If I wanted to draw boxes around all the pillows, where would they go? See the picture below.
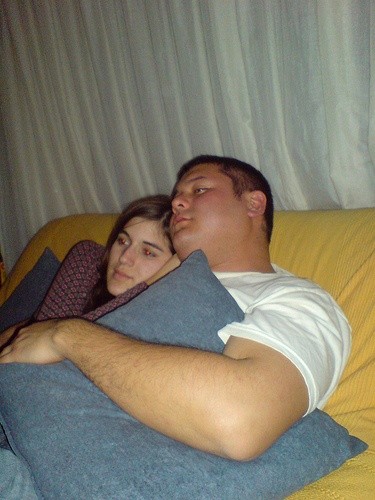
[0,249,369,500]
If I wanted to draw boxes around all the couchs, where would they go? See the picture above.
[1,206,374,498]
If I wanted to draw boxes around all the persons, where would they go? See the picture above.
[0,193,182,357]
[0,152,351,500]
